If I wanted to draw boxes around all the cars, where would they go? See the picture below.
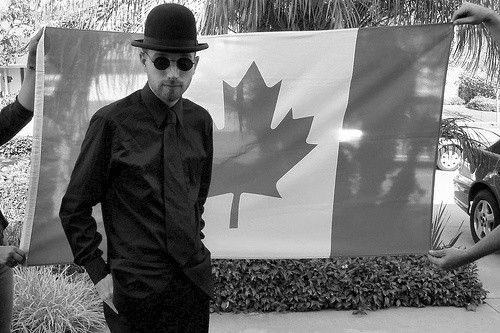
[433,111,500,172]
[452,136,499,244]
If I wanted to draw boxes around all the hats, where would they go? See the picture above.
[131,3,209,53]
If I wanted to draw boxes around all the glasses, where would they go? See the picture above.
[143,51,196,72]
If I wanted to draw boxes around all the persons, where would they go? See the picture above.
[429,2,500,270]
[0,27,45,333]
[60,3,214,333]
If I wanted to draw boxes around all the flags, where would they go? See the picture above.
[18,22,454,266]
